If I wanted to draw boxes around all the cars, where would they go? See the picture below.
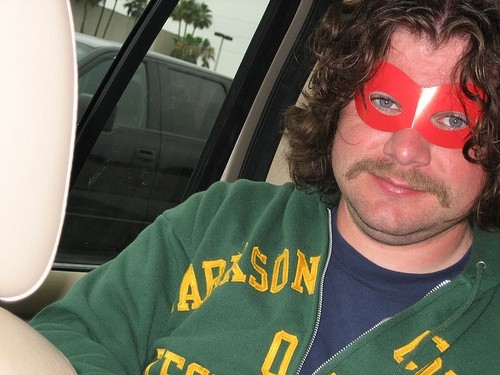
[54,29,236,269]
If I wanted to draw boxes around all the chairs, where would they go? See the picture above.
[0,0,80,374]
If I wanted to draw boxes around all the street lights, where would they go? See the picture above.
[213,32,233,71]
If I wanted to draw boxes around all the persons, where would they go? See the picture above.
[24,0,500,375]
